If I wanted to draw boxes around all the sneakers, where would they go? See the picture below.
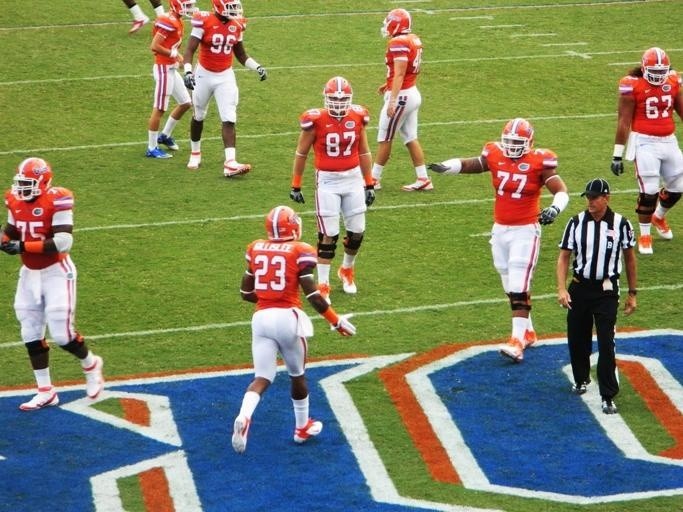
[289,417,326,447]
[570,379,590,395]
[127,16,150,35]
[315,281,333,308]
[186,151,202,170]
[497,327,539,365]
[16,384,62,411]
[649,212,677,241]
[221,158,252,178]
[228,412,252,456]
[600,396,619,415]
[360,175,383,191]
[399,176,435,193]
[635,233,654,256]
[79,354,108,401]
[144,146,174,161]
[156,131,180,151]
[336,264,359,296]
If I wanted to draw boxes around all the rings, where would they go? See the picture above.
[560,304,563,307]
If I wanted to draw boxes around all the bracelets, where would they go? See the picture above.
[365,175,374,186]
[245,56,261,70]
[2,235,10,245]
[170,49,177,59]
[551,191,570,213]
[292,175,302,188]
[439,158,462,176]
[23,241,43,254]
[628,290,637,295]
[321,307,339,326]
[184,63,193,76]
[612,144,626,158]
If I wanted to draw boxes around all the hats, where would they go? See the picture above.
[578,177,611,199]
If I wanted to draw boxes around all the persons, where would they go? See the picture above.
[123,0,164,34]
[183,1,268,178]
[555,179,638,414]
[232,206,356,454]
[0,157,104,412]
[611,47,683,256]
[144,1,195,159]
[289,75,375,306]
[364,9,435,192]
[428,118,570,361]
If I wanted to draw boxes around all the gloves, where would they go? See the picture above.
[255,64,268,82]
[608,155,628,179]
[535,204,561,229]
[286,186,307,205]
[329,315,357,337]
[426,159,452,178]
[175,51,183,67]
[0,235,24,256]
[364,184,376,207]
[183,71,196,91]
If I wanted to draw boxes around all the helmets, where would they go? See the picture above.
[17,156,54,197]
[640,46,672,79]
[263,204,302,243]
[381,7,414,37]
[500,116,537,154]
[320,75,355,100]
[210,0,241,17]
[168,0,196,17]
